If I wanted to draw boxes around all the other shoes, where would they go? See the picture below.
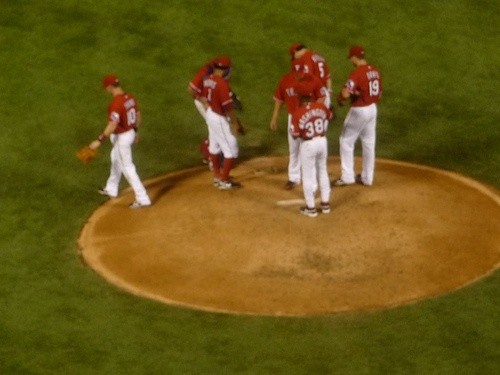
[214,178,241,189]
[334,178,347,185]
[301,205,319,217]
[359,174,362,180]
[130,203,148,208]
[286,181,293,190]
[321,204,330,214]
[99,189,118,198]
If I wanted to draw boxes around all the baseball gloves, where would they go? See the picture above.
[75,145,97,163]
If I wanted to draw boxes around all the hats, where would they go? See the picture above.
[292,59,311,77]
[103,76,118,89]
[216,55,231,68]
[289,43,303,62]
[348,48,365,59]
[298,82,314,96]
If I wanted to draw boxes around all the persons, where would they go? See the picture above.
[77,76,151,210]
[337,46,382,186]
[188,55,248,190]
[271,43,333,217]
